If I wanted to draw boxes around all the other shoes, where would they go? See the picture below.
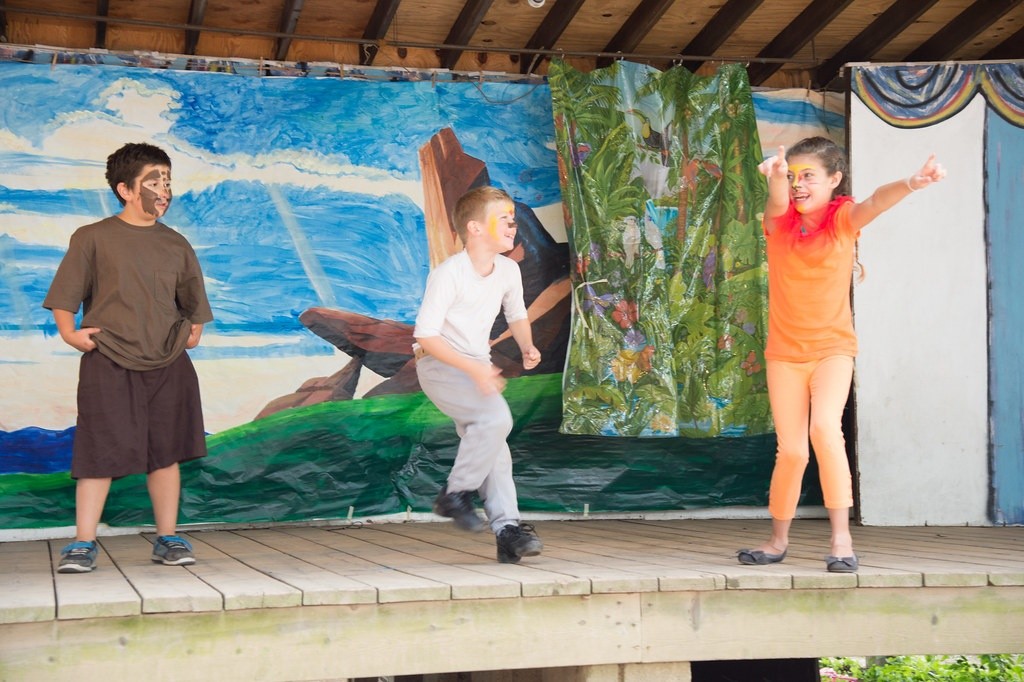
[736,546,787,565]
[824,552,859,573]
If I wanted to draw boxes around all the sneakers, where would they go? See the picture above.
[435,485,486,534]
[57,541,97,573]
[496,523,544,563]
[151,535,196,565]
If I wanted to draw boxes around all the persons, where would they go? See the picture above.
[737,135,946,572]
[412,186,541,564]
[42,143,215,573]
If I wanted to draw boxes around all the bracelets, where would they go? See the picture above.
[907,174,927,195]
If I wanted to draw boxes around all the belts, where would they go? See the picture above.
[414,347,430,360]
